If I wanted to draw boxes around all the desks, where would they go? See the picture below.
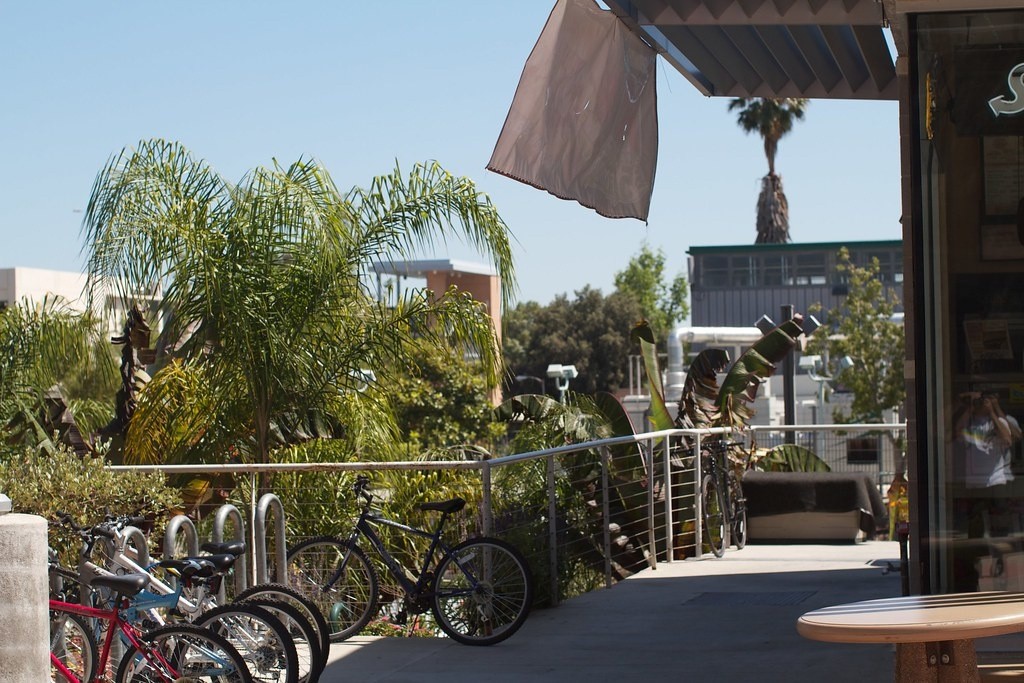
[797,591,1024,683]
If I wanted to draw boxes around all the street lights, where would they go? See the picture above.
[754,303,822,444]
[546,364,579,406]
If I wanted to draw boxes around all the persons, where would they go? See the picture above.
[955,390,1023,564]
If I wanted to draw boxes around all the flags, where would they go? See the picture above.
[485,0,658,227]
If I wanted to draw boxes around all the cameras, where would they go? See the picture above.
[973,397,983,406]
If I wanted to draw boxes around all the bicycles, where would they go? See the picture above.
[47,509,331,683]
[670,439,747,558]
[269,473,534,647]
